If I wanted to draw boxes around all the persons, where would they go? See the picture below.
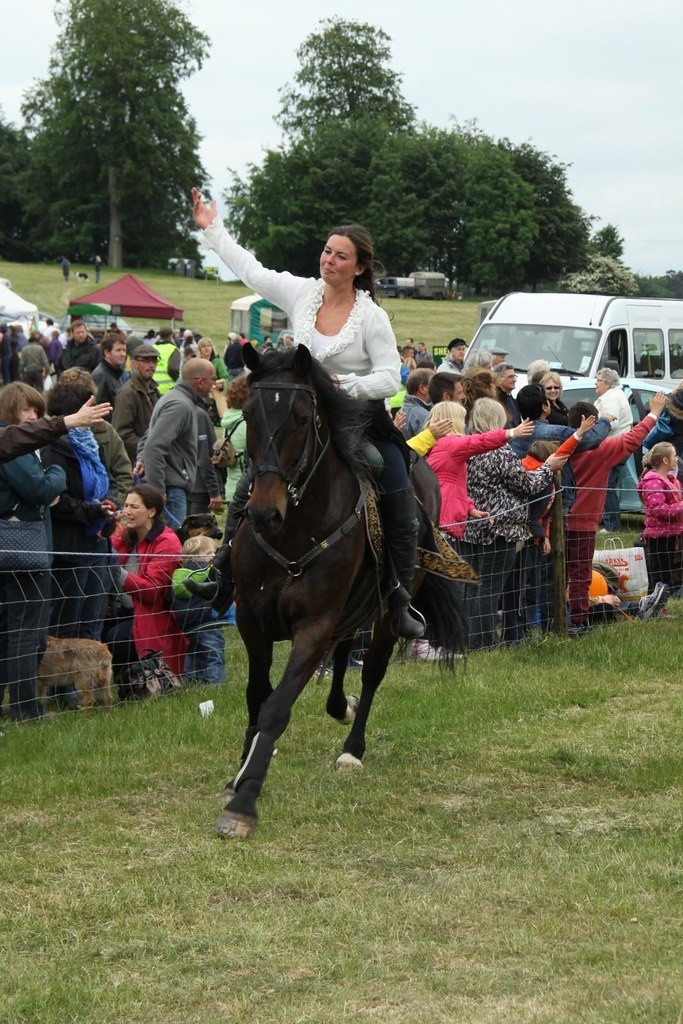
[95,254,101,283]
[0,316,683,722]
[182,184,426,639]
[60,258,70,280]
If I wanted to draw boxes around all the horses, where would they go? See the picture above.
[214,341,443,841]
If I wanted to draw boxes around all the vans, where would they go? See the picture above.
[408,272,446,299]
[460,292,683,387]
[374,278,415,300]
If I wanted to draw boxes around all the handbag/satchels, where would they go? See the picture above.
[592,537,649,602]
[641,404,672,450]
[0,519,49,569]
[119,648,181,700]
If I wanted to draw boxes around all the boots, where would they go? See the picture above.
[379,485,425,638]
[183,493,249,618]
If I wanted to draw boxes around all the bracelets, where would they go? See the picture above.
[510,427,515,440]
[573,432,582,441]
[648,412,658,421]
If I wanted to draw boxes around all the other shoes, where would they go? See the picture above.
[1,707,10,715]
[598,527,619,534]
[42,709,56,722]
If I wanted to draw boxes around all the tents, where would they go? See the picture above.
[66,274,184,329]
[230,292,294,350]
[0,277,38,316]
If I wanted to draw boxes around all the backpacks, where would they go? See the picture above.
[211,419,248,470]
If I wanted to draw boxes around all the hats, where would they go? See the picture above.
[447,339,465,351]
[488,347,509,355]
[132,344,160,358]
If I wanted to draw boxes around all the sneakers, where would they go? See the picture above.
[431,643,466,659]
[332,655,364,669]
[316,652,331,676]
[638,581,670,622]
[406,637,443,661]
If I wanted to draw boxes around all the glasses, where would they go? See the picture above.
[134,357,157,363]
[194,376,216,382]
[545,386,560,390]
[506,374,517,379]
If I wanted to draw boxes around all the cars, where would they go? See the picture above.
[0,312,134,344]
[556,378,672,514]
